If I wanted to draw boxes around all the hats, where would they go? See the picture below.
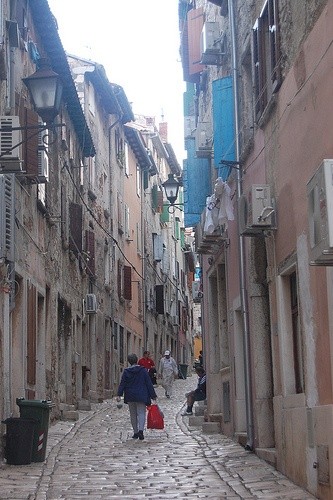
[164,350,170,355]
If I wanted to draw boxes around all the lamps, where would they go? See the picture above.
[163,173,183,213]
[0,55,65,156]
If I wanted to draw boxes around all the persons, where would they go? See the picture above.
[198,350,203,364]
[117,354,158,440]
[158,350,178,398]
[138,350,155,371]
[180,367,206,416]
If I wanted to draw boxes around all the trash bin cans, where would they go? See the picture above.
[1,418,40,464]
[195,363,200,368]
[16,396,50,462]
[178,364,188,379]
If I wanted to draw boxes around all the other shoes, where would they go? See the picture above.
[181,411,193,416]
[165,391,170,398]
[132,433,139,439]
[138,430,144,440]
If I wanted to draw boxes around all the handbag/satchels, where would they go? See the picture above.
[147,404,164,429]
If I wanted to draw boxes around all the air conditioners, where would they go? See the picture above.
[38,120,49,153]
[194,121,212,158]
[86,293,97,313]
[171,315,179,325]
[195,214,223,255]
[193,281,199,303]
[0,115,23,171]
[239,184,274,235]
[38,150,49,182]
[200,21,220,64]
[305,159,333,267]
